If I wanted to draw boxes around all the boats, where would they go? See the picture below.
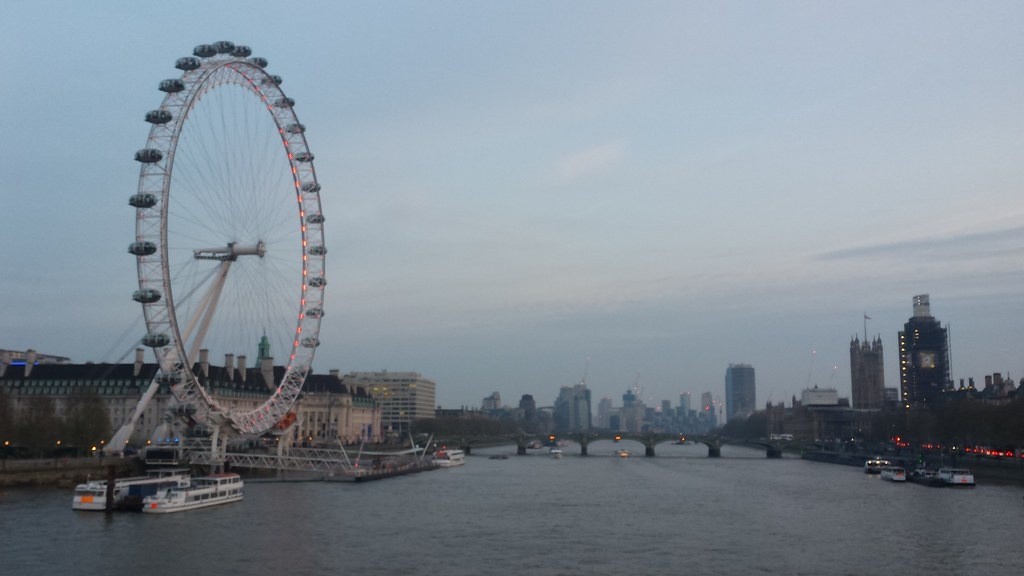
[617,449,631,458]
[142,474,245,513]
[549,447,562,458]
[908,467,977,487]
[864,457,891,473]
[430,449,464,467]
[71,468,191,510]
[880,463,906,482]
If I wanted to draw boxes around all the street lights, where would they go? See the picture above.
[385,392,396,432]
